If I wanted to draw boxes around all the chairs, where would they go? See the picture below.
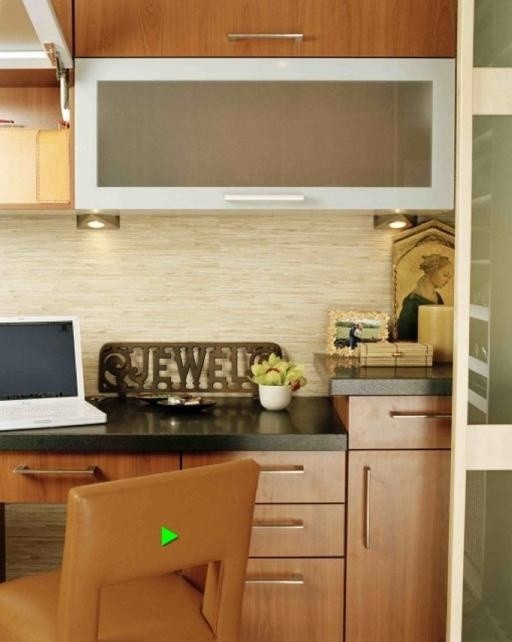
[0,459,261,641]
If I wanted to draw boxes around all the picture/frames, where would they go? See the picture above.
[327,309,389,357]
[392,218,455,341]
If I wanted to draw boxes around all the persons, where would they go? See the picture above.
[349,324,363,348]
[398,253,453,340]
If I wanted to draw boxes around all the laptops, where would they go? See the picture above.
[0,316,107,431]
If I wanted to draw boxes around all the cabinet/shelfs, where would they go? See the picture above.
[0,452,182,504]
[1,2,457,214]
[333,394,452,641]
[181,451,346,641]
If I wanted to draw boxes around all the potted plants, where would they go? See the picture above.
[248,352,308,411]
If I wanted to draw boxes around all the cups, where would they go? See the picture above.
[259,413,289,432]
[148,394,212,410]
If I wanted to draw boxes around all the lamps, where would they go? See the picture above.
[373,214,417,231]
[77,214,121,230]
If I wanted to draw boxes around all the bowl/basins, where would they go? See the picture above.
[259,385,293,410]
